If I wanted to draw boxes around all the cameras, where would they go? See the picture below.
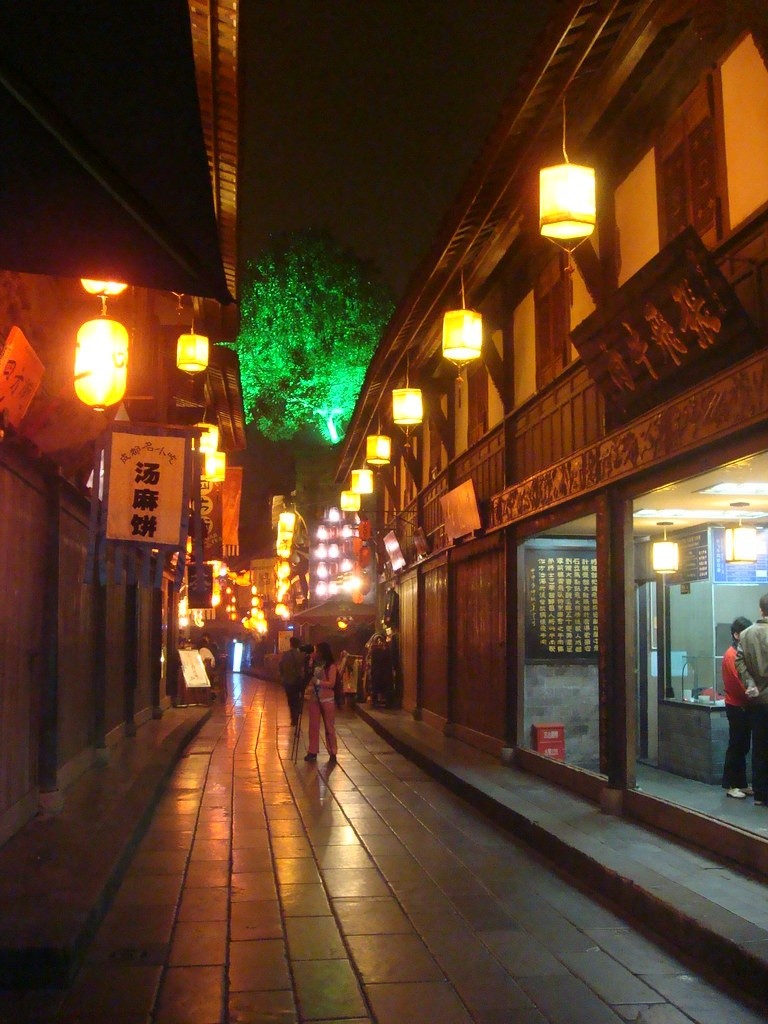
[300,644,314,654]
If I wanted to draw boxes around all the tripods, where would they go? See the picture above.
[291,654,336,765]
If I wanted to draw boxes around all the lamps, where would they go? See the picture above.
[441,270,483,406]
[350,470,373,506]
[366,411,391,485]
[652,522,680,575]
[393,354,423,474]
[725,504,759,565]
[340,490,361,523]
[176,318,208,377]
[191,407,220,454]
[539,94,595,306]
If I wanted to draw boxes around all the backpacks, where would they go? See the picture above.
[326,664,346,706]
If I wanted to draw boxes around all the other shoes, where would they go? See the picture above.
[754,801,764,805]
[330,754,337,762]
[726,786,753,799]
[303,752,318,762]
[290,720,298,726]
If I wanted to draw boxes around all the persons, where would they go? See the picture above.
[721,592,768,806]
[279,637,305,726]
[304,642,337,764]
[199,632,219,668]
